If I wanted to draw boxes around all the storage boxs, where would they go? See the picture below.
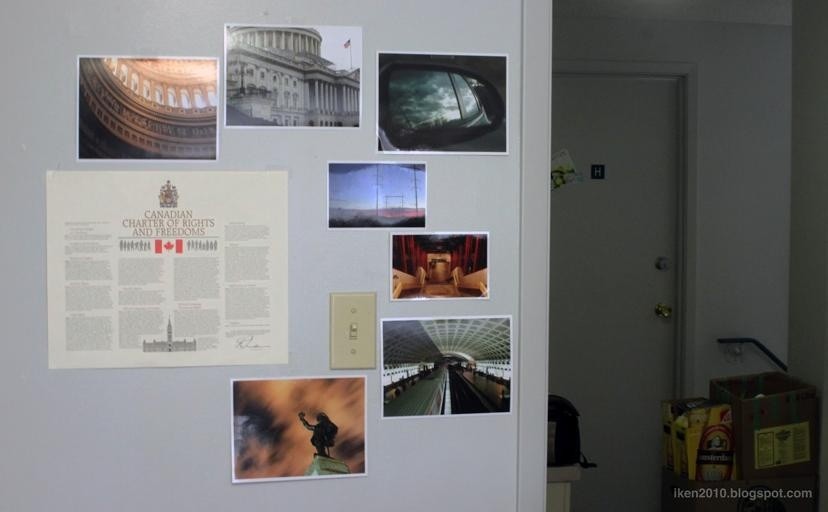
[707,371,821,481]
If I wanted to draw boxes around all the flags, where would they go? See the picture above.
[344,40,351,50]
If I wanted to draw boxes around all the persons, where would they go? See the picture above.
[299,411,338,457]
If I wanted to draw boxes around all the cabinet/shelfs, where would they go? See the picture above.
[546,464,580,512]
